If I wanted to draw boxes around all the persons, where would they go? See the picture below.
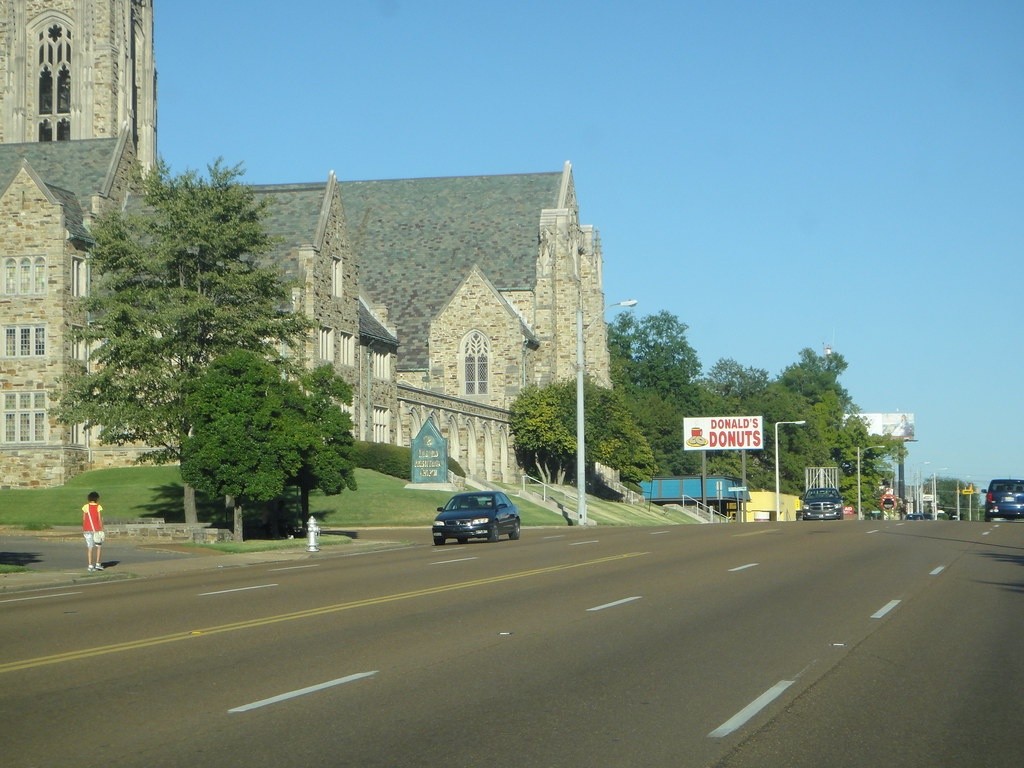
[79,492,106,573]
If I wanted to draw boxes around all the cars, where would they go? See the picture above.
[923,513,934,520]
[799,488,845,520]
[981,479,1024,522]
[432,491,520,545]
[905,514,924,520]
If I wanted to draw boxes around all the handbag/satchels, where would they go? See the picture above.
[93,531,105,543]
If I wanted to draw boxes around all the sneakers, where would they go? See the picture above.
[95,563,104,571]
[87,564,98,573]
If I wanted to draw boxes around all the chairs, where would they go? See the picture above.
[460,498,478,509]
[997,486,1003,492]
[1016,487,1023,492]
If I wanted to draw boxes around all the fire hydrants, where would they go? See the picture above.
[307,516,321,552]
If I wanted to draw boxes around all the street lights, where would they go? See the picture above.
[577,300,638,526]
[775,420,806,522]
[857,446,885,520]
[909,462,930,513]
